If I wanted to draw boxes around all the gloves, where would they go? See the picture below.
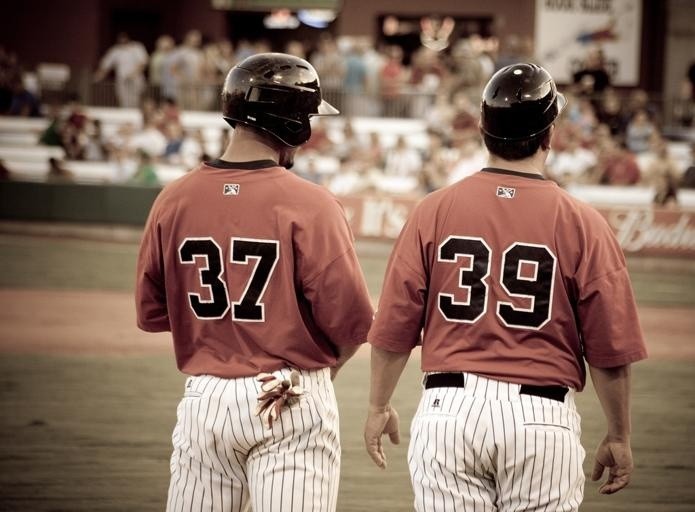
[254,371,302,430]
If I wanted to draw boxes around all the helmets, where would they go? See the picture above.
[480,64,566,139]
[222,53,340,147]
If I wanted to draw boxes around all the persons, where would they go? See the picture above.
[132,51,377,511]
[364,61,649,512]
